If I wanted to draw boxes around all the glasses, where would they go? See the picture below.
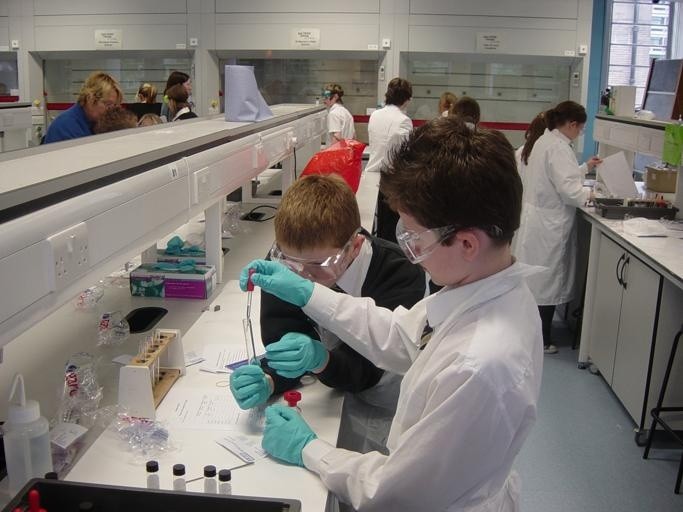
[270,227,367,285]
[395,217,459,265]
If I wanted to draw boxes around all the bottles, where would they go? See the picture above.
[143,460,232,497]
[2,370,52,499]
[244,317,256,369]
[135,329,160,391]
[315,98,319,105]
[599,89,610,114]
[621,193,673,210]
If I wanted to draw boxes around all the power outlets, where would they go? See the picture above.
[44,217,93,290]
[285,131,296,154]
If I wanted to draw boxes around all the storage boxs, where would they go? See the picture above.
[642,166,678,195]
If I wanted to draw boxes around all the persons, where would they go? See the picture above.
[90,70,197,132]
[447,96,480,127]
[320,81,356,147]
[239,114,545,512]
[511,99,602,355]
[41,70,122,143]
[438,92,456,116]
[228,172,426,412]
[363,77,414,173]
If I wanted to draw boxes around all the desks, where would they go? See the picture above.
[587,202,683,446]
[0,139,386,512]
[573,193,594,221]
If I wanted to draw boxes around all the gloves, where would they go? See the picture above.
[229,365,272,410]
[240,259,315,308]
[264,331,328,379]
[262,403,320,467]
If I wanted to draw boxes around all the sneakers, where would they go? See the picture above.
[544,344,557,354]
